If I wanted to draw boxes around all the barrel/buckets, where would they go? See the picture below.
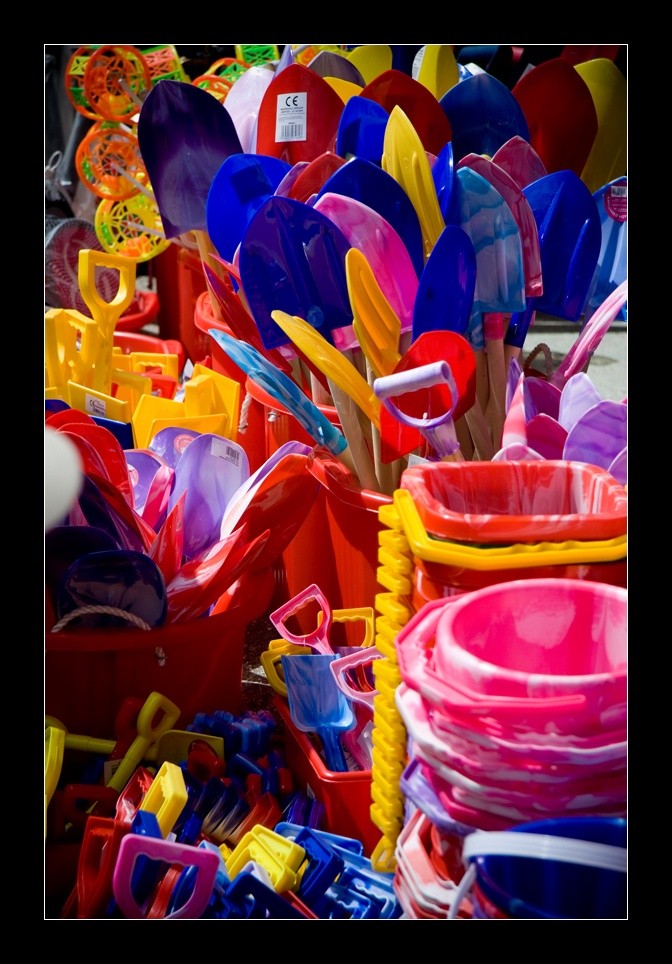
[45,291,627,921]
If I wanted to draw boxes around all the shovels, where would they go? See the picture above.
[134,43,630,501]
[44,402,323,635]
[280,652,357,774]
